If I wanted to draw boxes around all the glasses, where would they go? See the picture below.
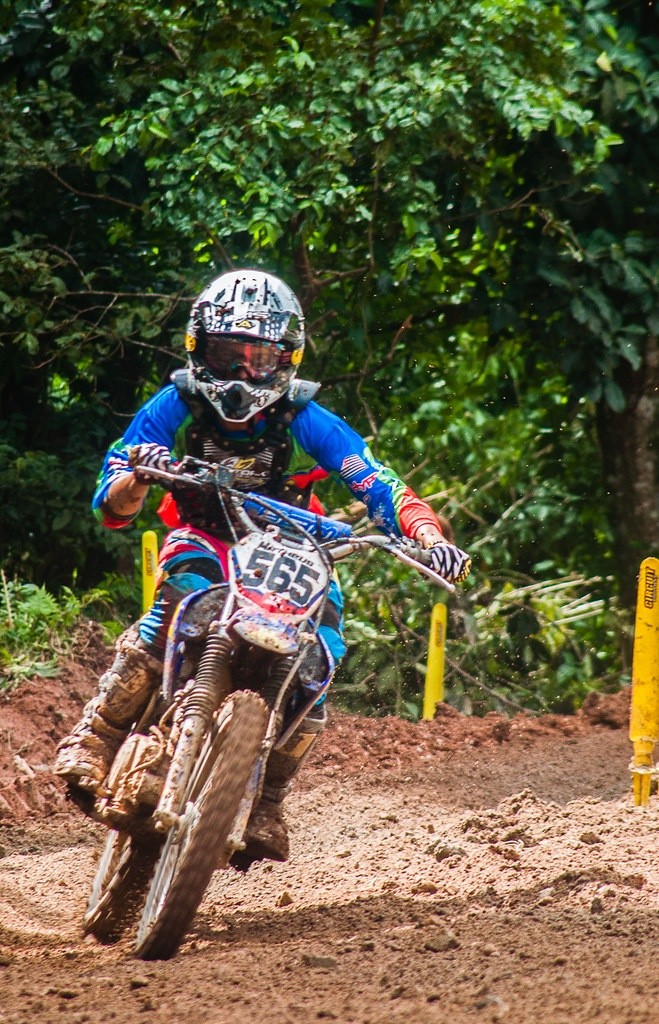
[184,334,303,383]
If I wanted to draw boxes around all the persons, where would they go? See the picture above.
[55,268,476,861]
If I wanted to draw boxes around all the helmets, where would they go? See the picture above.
[183,270,306,422]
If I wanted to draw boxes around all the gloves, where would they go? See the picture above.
[127,443,172,484]
[420,542,472,584]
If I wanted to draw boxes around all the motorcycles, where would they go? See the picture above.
[62,447,455,959]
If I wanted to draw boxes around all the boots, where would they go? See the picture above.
[53,620,164,787]
[241,705,327,862]
